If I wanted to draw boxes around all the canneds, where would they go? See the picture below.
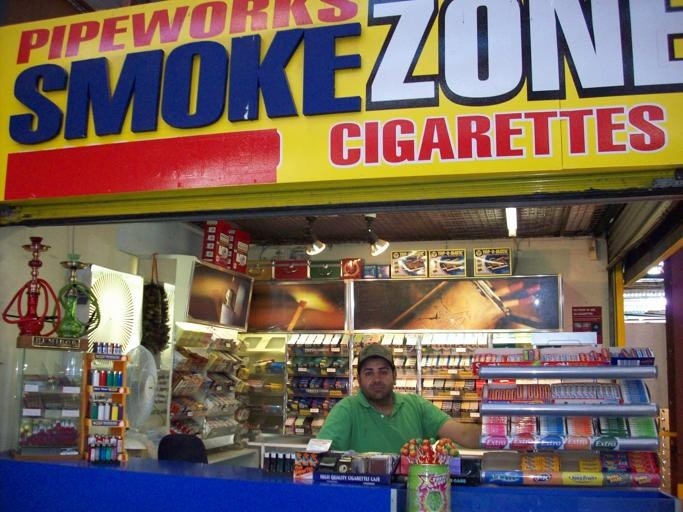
[94,342,121,355]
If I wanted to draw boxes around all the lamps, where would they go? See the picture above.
[301,212,326,255]
[363,213,390,257]
[504,205,518,238]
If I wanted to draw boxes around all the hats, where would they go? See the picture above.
[357,342,395,368]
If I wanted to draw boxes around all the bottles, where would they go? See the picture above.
[218,287,235,323]
[87,368,123,463]
[90,340,121,355]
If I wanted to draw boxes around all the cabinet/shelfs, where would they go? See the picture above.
[13,348,130,471]
[172,323,244,450]
[474,360,663,485]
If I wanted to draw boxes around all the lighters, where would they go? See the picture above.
[86,341,127,464]
[264,454,296,474]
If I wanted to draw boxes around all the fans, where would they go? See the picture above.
[122,345,158,449]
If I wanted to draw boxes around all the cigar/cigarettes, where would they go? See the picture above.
[498,281,544,308]
[286,300,309,333]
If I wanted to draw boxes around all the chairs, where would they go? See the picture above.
[157,431,211,464]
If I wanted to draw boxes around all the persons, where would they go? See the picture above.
[315,343,482,456]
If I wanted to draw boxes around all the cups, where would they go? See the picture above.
[386,277,508,331]
